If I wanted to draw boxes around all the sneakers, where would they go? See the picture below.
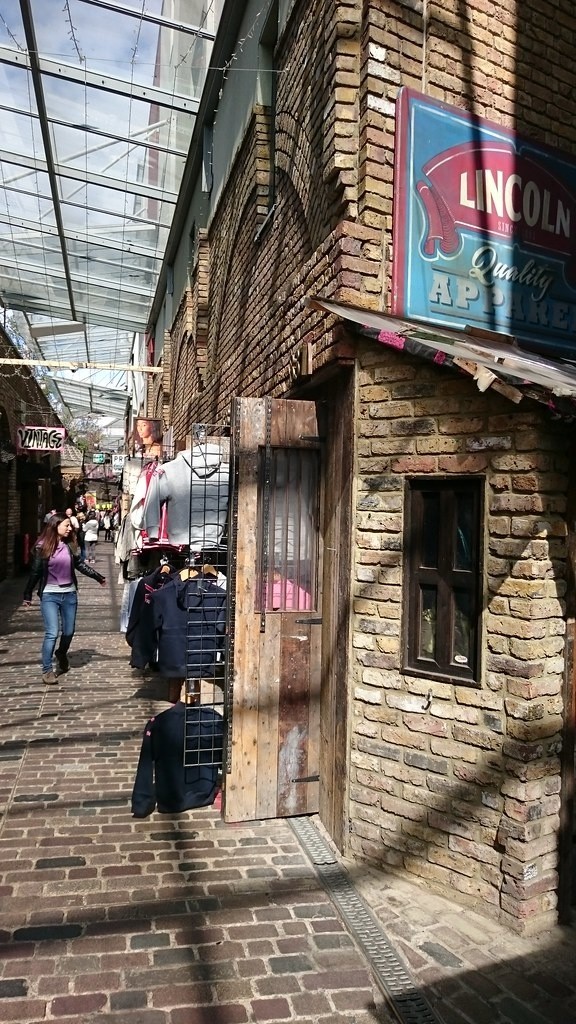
[56,650,70,670]
[43,671,59,684]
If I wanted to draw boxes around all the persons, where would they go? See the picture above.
[22,510,107,683]
[64,503,122,563]
[135,419,161,458]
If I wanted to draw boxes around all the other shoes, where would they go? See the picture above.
[84,559,89,563]
[90,560,95,564]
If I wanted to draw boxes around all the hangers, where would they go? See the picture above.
[180,694,196,704]
[198,554,220,580]
[179,554,199,583]
[159,555,170,575]
